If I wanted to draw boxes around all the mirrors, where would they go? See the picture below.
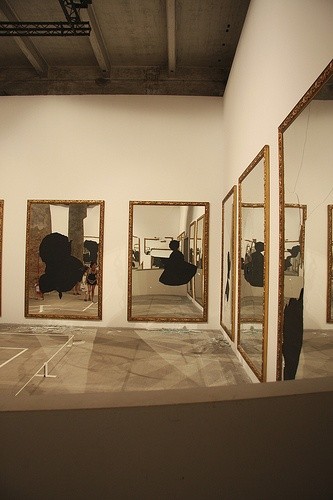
[219,184,235,343]
[275,56,332,382]
[236,144,268,384]
[240,202,264,324]
[283,203,307,301]
[132,213,204,310]
[23,199,104,321]
[127,200,210,323]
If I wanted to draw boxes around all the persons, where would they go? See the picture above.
[71,279,81,296]
[85,261,99,301]
